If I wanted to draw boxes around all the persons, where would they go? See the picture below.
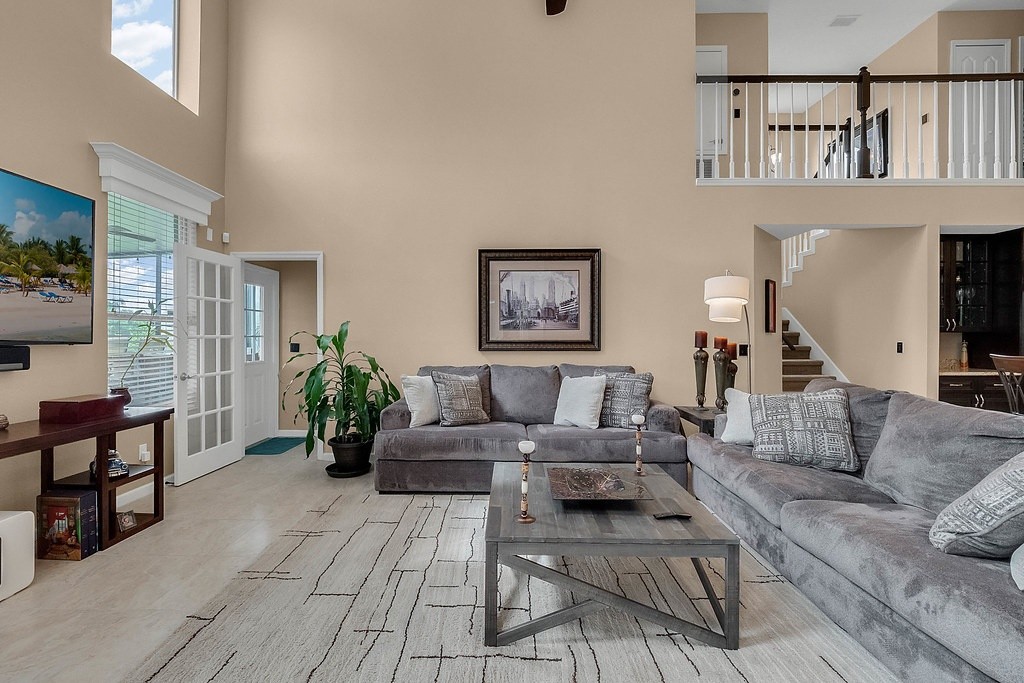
[62,276,67,283]
[50,277,54,283]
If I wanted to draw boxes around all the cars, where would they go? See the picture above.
[43,279,54,284]
[0,278,11,284]
[89,452,129,478]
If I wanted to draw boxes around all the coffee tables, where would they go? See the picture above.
[484,460,745,651]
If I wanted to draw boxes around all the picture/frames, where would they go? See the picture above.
[477,248,602,351]
[765,278,777,333]
[115,510,138,533]
[826,109,890,178]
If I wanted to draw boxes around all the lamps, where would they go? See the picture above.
[704,270,752,394]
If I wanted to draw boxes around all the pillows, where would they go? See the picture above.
[594,369,655,430]
[401,373,440,428]
[721,387,756,445]
[430,368,491,426]
[552,375,607,428]
[930,450,1024,559]
[748,387,861,471]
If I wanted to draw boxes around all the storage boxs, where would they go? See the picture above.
[0,508,36,603]
[34,493,97,561]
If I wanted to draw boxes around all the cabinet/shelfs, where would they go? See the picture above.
[0,404,177,548]
[939,374,1024,412]
[940,236,994,334]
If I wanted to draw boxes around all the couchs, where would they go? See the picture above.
[372,363,692,494]
[685,379,1024,683]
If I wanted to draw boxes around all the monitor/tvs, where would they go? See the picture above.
[0,169,96,344]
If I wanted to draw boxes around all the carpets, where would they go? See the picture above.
[245,436,307,456]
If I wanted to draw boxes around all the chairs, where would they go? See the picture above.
[990,352,1024,417]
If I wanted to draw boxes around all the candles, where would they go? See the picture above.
[694,329,708,347]
[725,341,737,358]
[713,335,727,347]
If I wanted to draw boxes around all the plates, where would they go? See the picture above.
[548,467,654,500]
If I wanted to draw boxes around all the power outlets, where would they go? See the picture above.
[138,443,148,460]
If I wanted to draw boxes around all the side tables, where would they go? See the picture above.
[674,404,727,496]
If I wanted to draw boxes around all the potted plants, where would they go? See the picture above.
[111,292,187,406]
[276,319,400,467]
